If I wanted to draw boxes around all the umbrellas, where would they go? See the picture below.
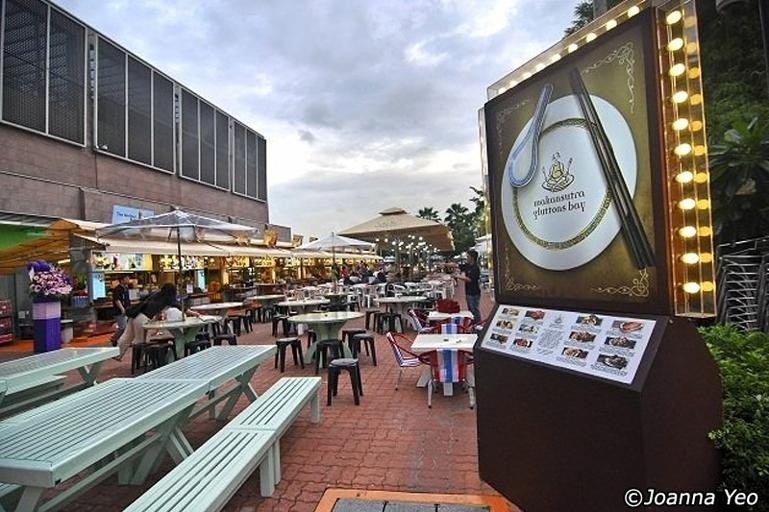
[291,234,372,283]
[336,207,449,280]
[426,231,454,244]
[438,241,455,251]
[454,253,468,260]
[95,209,260,320]
[475,234,491,242]
[470,243,485,254]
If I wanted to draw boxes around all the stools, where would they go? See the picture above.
[150,337,175,344]
[312,339,345,376]
[219,376,324,485]
[308,329,319,348]
[272,314,290,337]
[352,333,378,366]
[224,317,242,335]
[186,341,211,358]
[274,338,303,373]
[252,304,263,323]
[214,334,238,345]
[327,358,363,407]
[382,313,404,334]
[195,332,209,339]
[365,310,380,330]
[130,342,148,374]
[237,314,253,333]
[374,312,385,332]
[263,305,276,324]
[343,328,366,351]
[150,344,177,369]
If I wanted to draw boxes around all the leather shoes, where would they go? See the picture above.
[111,339,116,346]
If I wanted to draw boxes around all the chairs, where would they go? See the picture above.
[318,293,349,309]
[427,348,476,411]
[387,332,430,391]
[430,324,470,334]
[409,309,436,334]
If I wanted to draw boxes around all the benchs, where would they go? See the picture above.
[120,429,274,512]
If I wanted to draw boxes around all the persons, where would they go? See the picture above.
[450,249,482,325]
[359,264,369,274]
[341,266,350,278]
[361,269,374,283]
[331,263,340,283]
[342,258,348,266]
[377,268,386,283]
[343,276,355,291]
[111,282,200,362]
[109,275,131,347]
[357,260,365,271]
